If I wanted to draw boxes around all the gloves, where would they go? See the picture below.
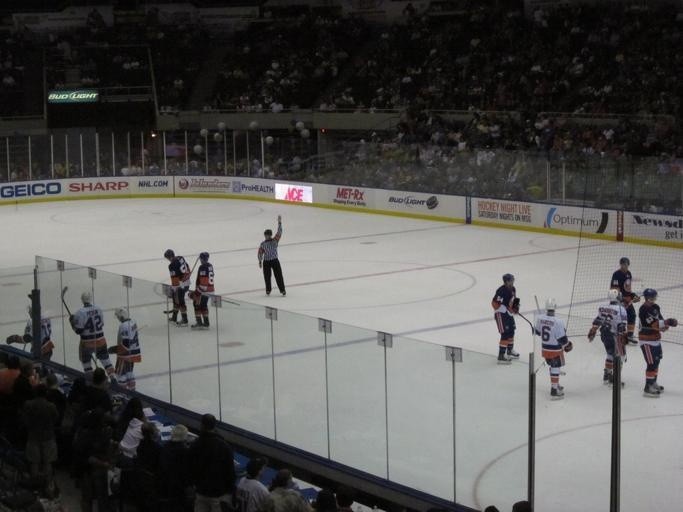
[588,327,596,342]
[658,317,678,331]
[564,341,572,352]
[6,334,20,344]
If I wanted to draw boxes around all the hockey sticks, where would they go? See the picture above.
[163,256,199,314]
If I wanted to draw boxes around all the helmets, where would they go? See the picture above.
[641,288,657,297]
[115,305,129,320]
[620,257,630,265]
[544,296,557,310]
[164,249,175,257]
[264,229,272,236]
[502,273,514,281]
[81,291,92,303]
[200,252,209,259]
[607,288,618,299]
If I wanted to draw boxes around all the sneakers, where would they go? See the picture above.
[608,368,624,387]
[110,374,118,383]
[557,384,564,391]
[176,314,188,324]
[191,320,202,328]
[654,378,664,393]
[496,346,512,361]
[550,387,565,396]
[507,344,520,356]
[644,379,660,394]
[603,370,613,382]
[266,288,271,293]
[281,289,286,295]
[169,311,177,322]
[202,317,210,328]
[628,332,637,344]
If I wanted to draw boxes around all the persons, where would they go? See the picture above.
[608,256,638,344]
[0,0,681,214]
[492,273,524,363]
[638,287,678,396]
[257,215,288,297]
[588,287,627,385]
[0,346,535,512]
[163,247,191,326]
[188,252,215,328]
[106,305,141,392]
[535,297,572,397]
[68,288,116,383]
[4,302,53,358]
